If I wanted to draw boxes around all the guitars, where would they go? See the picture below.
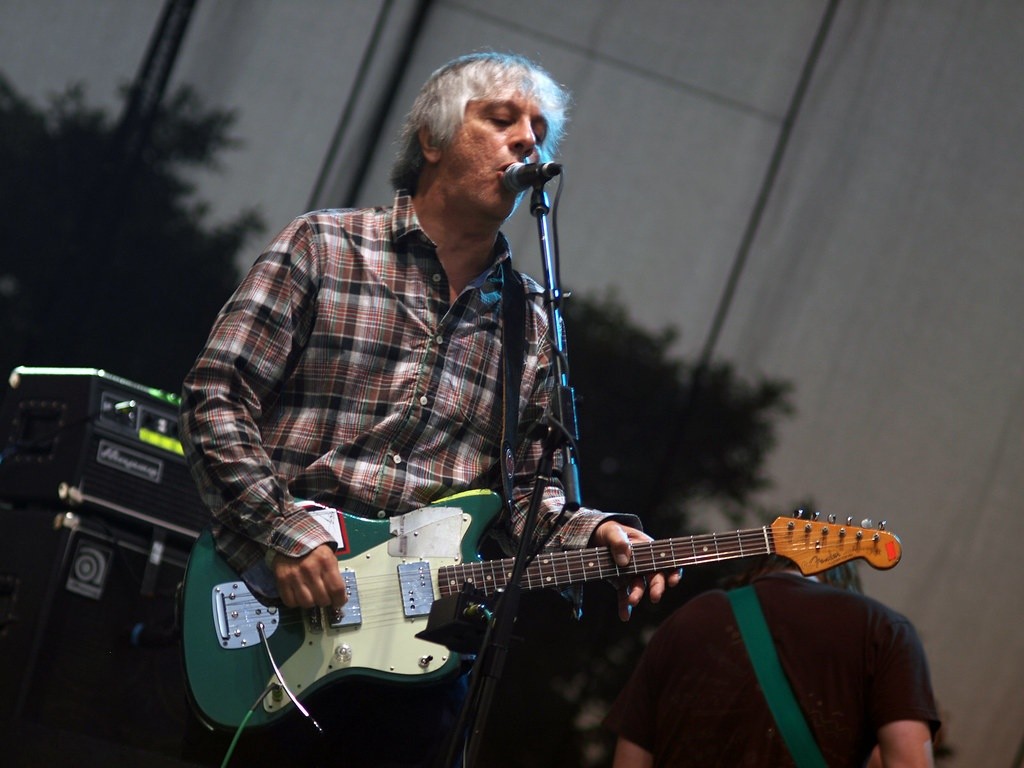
[182,486,904,728]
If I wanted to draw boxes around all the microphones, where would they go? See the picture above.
[504,160,562,193]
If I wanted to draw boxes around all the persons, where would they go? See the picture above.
[177,52,683,768]
[611,512,933,768]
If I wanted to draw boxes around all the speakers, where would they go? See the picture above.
[0,502,226,768]
